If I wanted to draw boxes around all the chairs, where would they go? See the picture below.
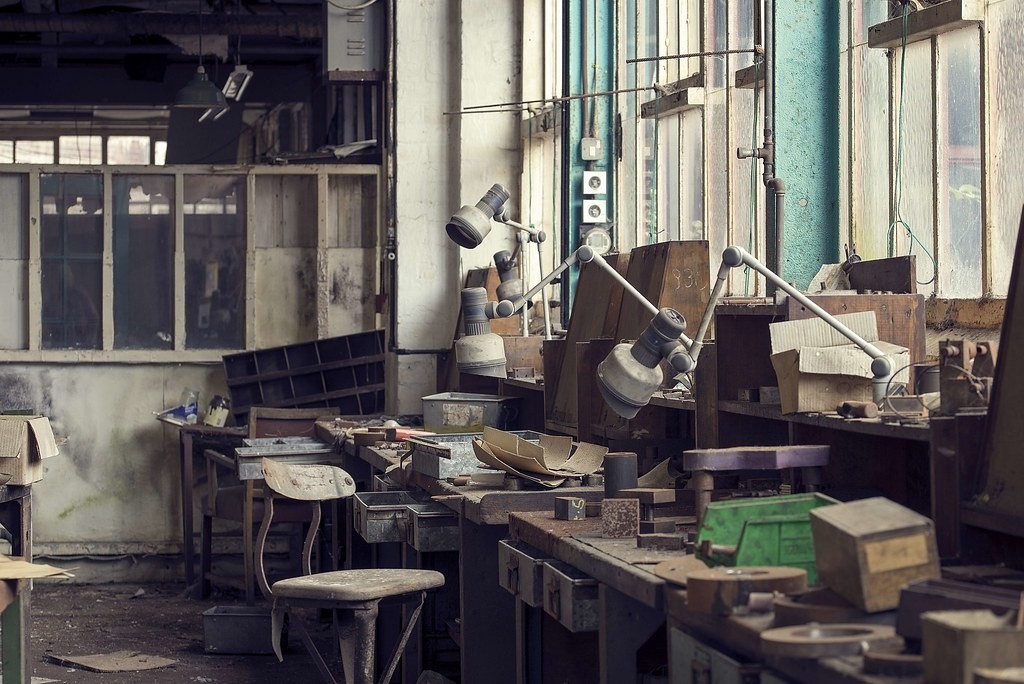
[198,406,343,601]
[255,457,446,684]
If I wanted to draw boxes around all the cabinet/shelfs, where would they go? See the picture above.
[498,491,1023,684]
[314,415,681,682]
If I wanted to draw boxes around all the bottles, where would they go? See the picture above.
[203,394,231,427]
[174,386,201,423]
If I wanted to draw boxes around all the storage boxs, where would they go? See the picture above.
[201,605,289,655]
[810,498,945,615]
[1,414,58,486]
[768,310,911,415]
[424,391,517,432]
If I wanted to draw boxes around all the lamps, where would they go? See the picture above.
[493,230,531,337]
[444,182,553,354]
[456,246,702,380]
[596,245,894,418]
[174,0,230,109]
